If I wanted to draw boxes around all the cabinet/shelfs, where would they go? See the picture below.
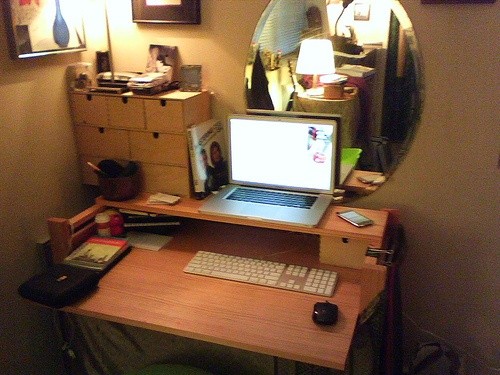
[69,88,209,198]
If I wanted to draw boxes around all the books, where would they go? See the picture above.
[62,236,131,274]
[121,208,181,228]
[187,117,228,194]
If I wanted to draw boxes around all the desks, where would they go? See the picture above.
[46,198,398,375]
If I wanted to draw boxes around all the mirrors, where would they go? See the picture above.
[242,0,424,204]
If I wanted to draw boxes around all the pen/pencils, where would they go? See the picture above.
[87,162,107,175]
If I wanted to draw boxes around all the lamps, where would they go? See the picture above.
[329,0,356,38]
[295,39,336,95]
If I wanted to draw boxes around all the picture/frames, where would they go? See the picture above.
[4,0,87,59]
[130,0,200,24]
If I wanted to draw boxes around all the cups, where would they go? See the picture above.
[323,85,344,99]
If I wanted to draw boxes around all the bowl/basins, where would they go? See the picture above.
[94,159,141,201]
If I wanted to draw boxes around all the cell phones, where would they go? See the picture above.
[357,173,381,185]
[336,209,374,229]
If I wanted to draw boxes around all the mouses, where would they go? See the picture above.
[311,300,338,326]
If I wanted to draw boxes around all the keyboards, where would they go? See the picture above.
[182,249,339,298]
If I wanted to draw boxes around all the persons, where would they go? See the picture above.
[209,140,227,186]
[197,148,218,191]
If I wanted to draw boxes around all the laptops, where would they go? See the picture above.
[197,114,341,228]
[248,109,363,183]
